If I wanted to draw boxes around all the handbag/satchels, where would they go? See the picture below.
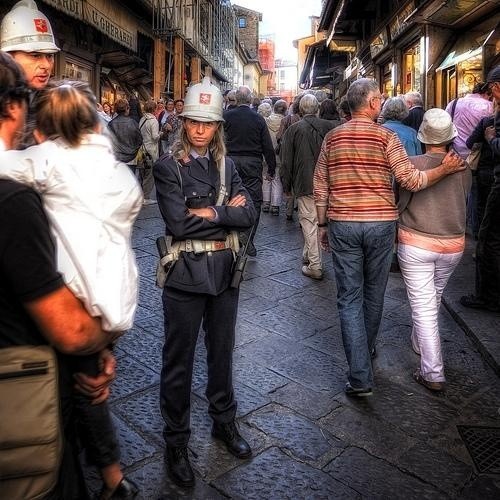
[0,345,63,499]
[136,153,153,169]
[465,142,483,171]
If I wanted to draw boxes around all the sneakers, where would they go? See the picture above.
[143,199,157,206]
[262,202,298,220]
[460,294,498,314]
[344,382,373,397]
[413,368,442,390]
[302,266,322,279]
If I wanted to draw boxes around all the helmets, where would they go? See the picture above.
[1,1,62,54]
[179,76,226,123]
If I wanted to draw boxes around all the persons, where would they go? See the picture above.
[0,78,146,500]
[460,67,500,309]
[99,83,492,279]
[0,51,117,500]
[391,106,472,391]
[151,74,257,487]
[310,76,468,398]
[1,0,61,149]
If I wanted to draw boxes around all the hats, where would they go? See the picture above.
[416,108,459,146]
[481,67,500,89]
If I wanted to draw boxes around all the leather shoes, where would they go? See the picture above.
[211,421,252,459]
[108,478,139,500]
[165,446,198,488]
